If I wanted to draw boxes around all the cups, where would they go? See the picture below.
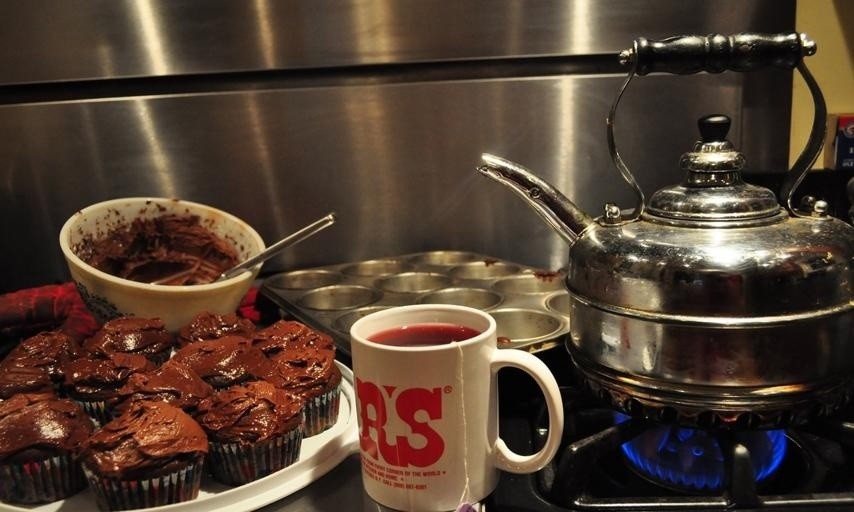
[350,301,563,512]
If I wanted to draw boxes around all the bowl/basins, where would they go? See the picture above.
[59,195,266,331]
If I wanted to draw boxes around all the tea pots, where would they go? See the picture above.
[474,32,854,421]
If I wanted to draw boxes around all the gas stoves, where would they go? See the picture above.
[521,384,854,512]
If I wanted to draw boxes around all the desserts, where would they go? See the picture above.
[0,308,343,511]
